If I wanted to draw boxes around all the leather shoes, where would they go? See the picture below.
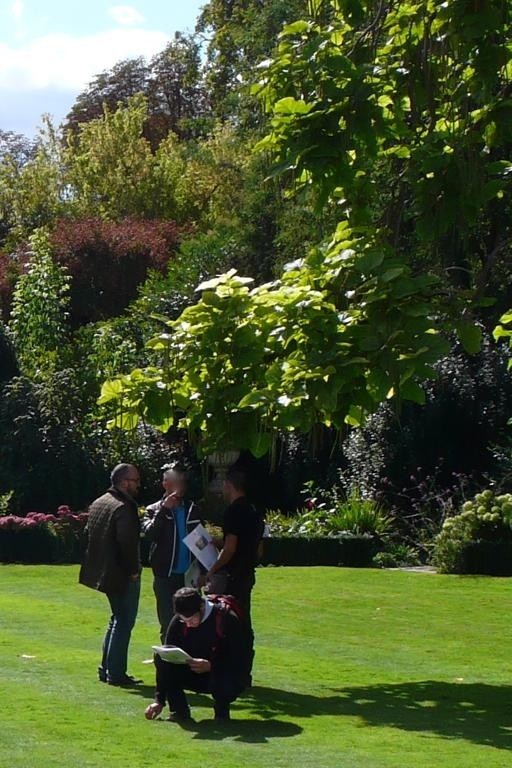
[98,668,144,686]
[165,708,192,721]
[214,712,231,721]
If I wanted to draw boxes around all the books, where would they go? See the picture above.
[182,522,229,581]
[184,558,204,590]
[150,643,193,665]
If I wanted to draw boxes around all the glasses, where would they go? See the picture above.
[179,612,199,624]
[125,476,140,483]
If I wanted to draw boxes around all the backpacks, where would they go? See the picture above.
[183,593,250,640]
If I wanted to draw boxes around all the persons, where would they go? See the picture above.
[143,586,254,721]
[76,462,145,687]
[190,447,268,690]
[145,467,207,642]
